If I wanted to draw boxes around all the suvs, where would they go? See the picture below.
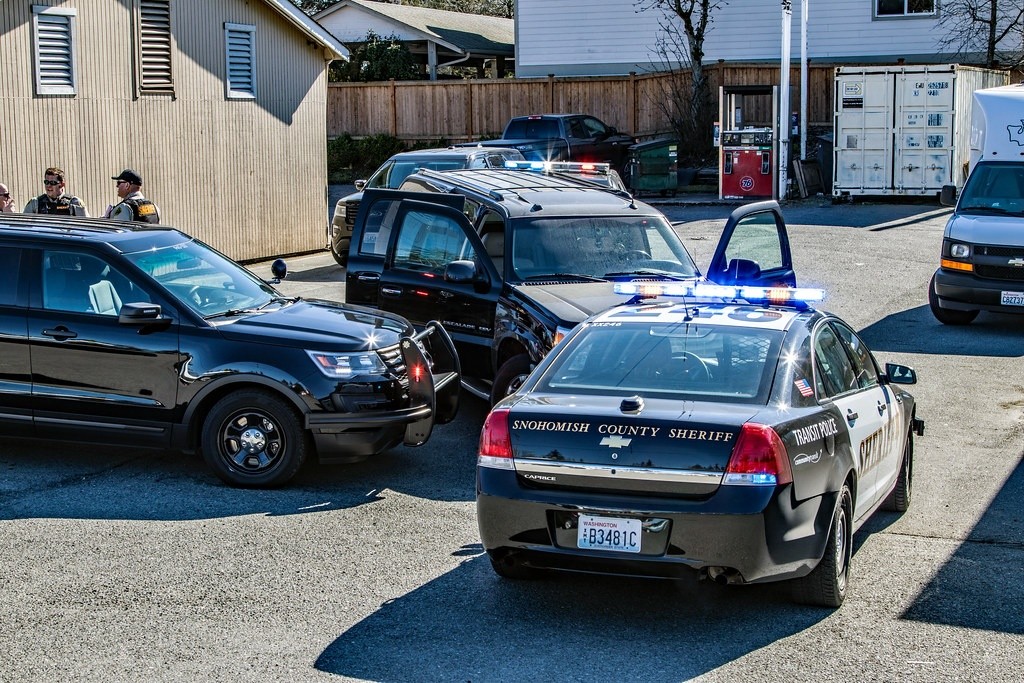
[0,209,464,491]
[345,167,799,410]
[328,141,534,268]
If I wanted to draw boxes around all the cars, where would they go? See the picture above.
[474,282,927,609]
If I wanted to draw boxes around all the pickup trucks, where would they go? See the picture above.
[449,114,641,189]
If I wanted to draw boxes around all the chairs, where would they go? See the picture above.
[481,233,504,274]
[986,169,1020,197]
[624,332,672,380]
[48,254,123,316]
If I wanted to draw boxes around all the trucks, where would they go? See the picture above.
[928,82,1024,326]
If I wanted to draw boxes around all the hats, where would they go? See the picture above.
[111,168,142,186]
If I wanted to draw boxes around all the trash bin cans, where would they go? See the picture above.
[629,138,679,198]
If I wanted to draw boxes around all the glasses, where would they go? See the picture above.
[0,193,9,198]
[117,180,127,185]
[44,180,62,185]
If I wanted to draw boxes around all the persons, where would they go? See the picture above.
[109,169,161,225]
[0,183,15,213]
[23,168,90,216]
